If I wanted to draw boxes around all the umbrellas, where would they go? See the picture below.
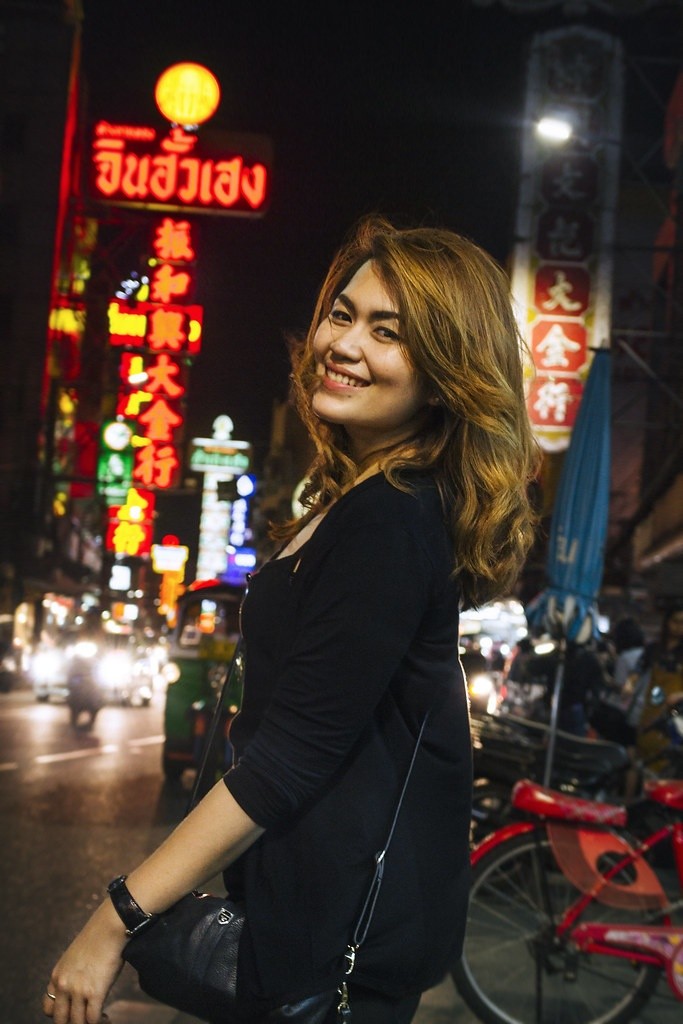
[540,341,612,789]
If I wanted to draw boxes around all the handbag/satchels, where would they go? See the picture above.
[122,891,354,1023]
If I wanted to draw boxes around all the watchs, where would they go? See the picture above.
[106,875,158,936]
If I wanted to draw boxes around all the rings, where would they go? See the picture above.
[47,992,55,999]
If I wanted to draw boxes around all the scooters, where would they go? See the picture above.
[57,626,118,728]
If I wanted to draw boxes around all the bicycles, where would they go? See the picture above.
[462,625,682,891]
[449,776,683,1024]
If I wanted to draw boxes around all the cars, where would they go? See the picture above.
[31,626,159,708]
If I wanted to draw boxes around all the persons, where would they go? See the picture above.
[41,219,545,1024]
